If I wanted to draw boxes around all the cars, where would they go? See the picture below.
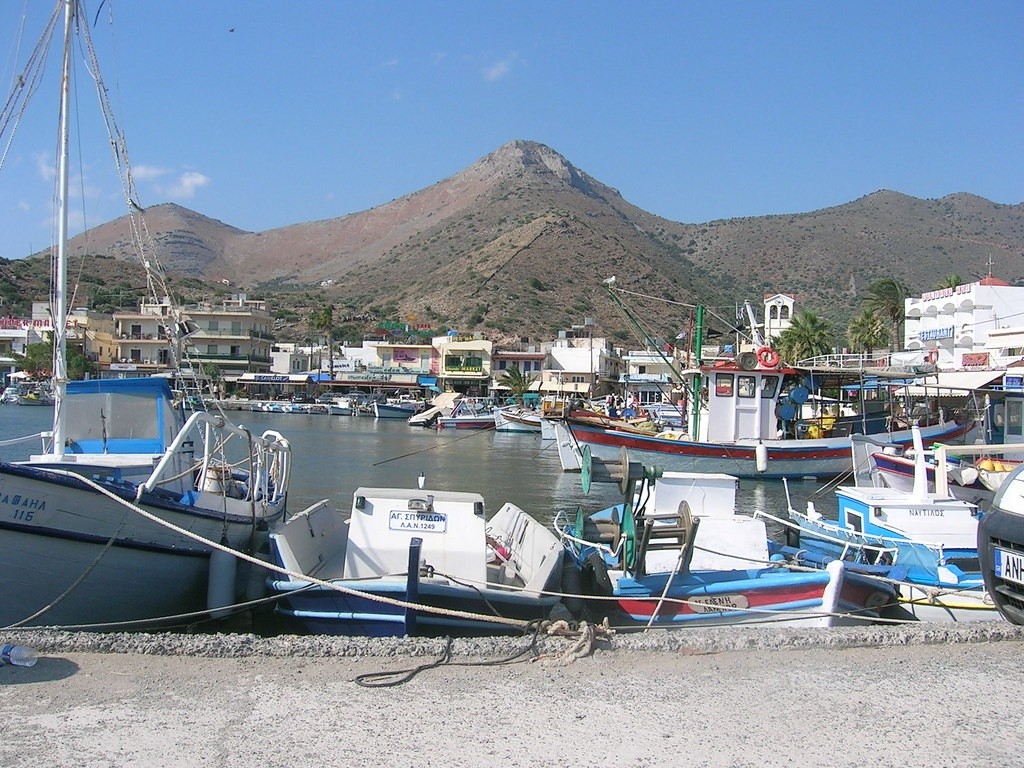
[976,460,1024,632]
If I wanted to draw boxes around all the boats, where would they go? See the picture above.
[551,444,903,628]
[265,468,568,637]
[1,343,1024,505]
[753,418,1006,622]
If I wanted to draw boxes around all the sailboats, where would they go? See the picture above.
[0,1,295,635]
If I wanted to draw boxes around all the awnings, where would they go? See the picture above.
[488,379,590,393]
[894,371,1006,397]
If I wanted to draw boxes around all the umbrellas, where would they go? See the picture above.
[7,371,30,378]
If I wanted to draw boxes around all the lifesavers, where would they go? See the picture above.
[757,347,779,367]
[928,348,940,364]
